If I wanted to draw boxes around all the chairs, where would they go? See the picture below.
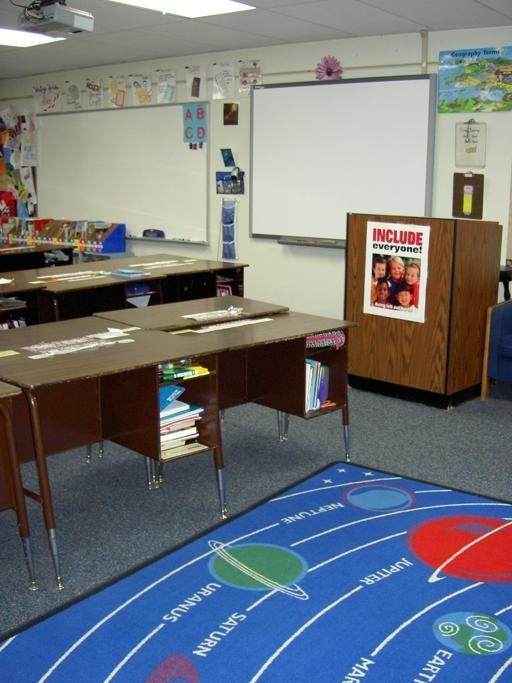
[481,298,511,402]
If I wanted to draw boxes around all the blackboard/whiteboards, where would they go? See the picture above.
[250,73,437,249]
[33,101,210,246]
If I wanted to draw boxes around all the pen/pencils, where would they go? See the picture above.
[311,239,337,243]
[279,239,306,241]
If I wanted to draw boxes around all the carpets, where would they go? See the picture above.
[0,460,512,683]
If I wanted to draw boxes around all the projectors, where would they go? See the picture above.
[17,3,95,40]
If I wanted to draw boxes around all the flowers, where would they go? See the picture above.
[315,55,343,80]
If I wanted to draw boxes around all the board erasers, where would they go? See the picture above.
[142,229,165,239]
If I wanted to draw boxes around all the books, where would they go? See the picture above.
[217,284,232,297]
[0,317,28,332]
[156,354,211,460]
[304,359,332,413]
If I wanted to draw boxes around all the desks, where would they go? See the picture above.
[1,380,43,591]
[0,315,141,366]
[2,330,230,590]
[154,309,359,512]
[95,294,289,331]
[1,235,250,328]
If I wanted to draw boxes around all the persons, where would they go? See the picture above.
[371,252,419,314]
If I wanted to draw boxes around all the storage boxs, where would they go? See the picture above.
[5,220,126,252]
